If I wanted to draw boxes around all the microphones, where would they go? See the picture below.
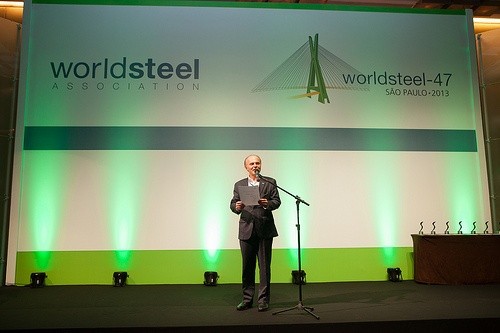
[254,171,261,177]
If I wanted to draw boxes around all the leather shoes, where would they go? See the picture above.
[258,303,269,311]
[236,301,253,311]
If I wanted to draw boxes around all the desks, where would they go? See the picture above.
[411,233,500,288]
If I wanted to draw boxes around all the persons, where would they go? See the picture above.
[230,154,282,312]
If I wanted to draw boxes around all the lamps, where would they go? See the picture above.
[113,271,129,287]
[29,272,47,288]
[387,267,403,283]
[291,269,308,286]
[203,270,219,287]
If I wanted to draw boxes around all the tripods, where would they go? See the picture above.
[260,175,320,320]
